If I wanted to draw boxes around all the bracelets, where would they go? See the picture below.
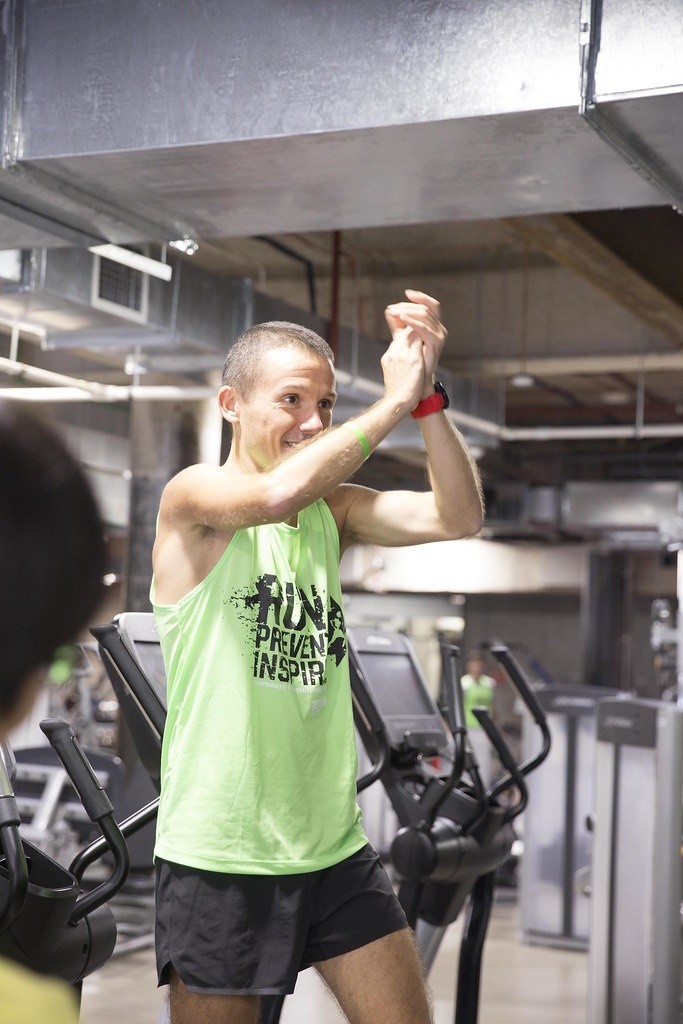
[341,420,370,460]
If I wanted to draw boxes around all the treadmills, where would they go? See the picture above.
[1,607,554,1024]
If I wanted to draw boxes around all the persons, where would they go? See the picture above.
[460,650,498,725]
[150,288,484,1024]
[0,400,117,1024]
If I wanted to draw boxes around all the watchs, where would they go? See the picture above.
[410,381,450,419]
